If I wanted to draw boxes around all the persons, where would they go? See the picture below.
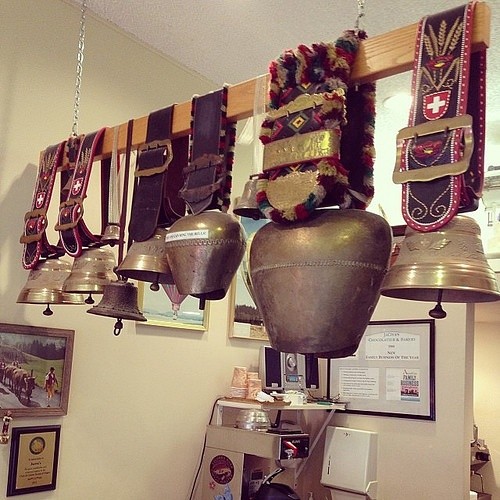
[2,410,14,436]
[44,366,58,408]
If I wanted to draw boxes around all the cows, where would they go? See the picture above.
[0,360,38,403]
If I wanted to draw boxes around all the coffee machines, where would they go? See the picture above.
[201,425,311,500]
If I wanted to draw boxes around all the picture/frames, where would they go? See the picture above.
[137,281,210,331]
[6,425,61,496]
[228,196,270,341]
[0,323,75,418]
[326,319,436,421]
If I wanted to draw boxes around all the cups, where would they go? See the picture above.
[291,395,304,406]
[226,366,261,401]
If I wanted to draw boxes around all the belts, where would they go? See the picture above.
[129,104,189,242]
